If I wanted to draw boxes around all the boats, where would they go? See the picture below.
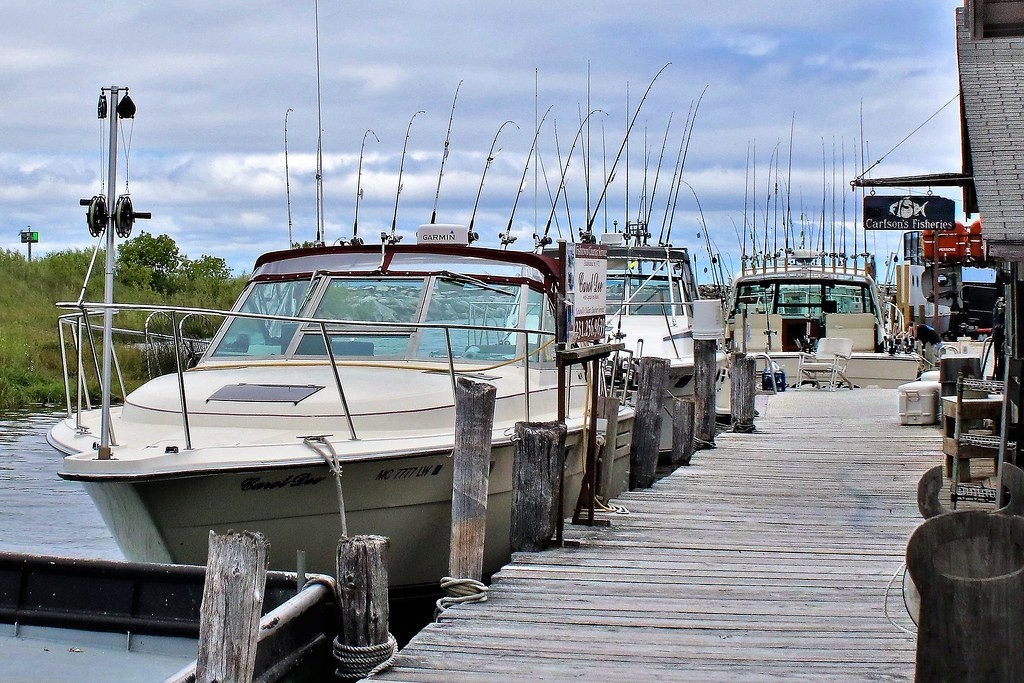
[44,1,898,612]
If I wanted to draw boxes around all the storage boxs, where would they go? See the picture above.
[897,380,941,426]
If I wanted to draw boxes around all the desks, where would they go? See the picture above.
[941,394,1003,482]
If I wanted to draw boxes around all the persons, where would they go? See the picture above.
[638,292,672,315]
[904,321,942,358]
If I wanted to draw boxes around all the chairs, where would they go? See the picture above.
[796,338,855,392]
